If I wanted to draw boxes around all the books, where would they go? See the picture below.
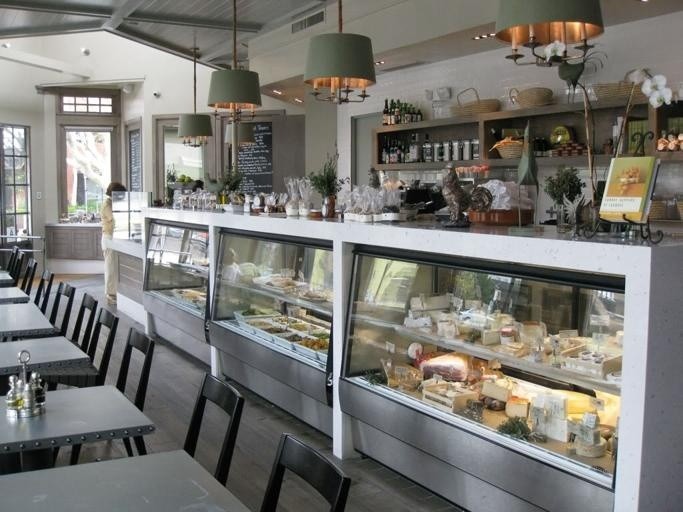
[598,157,662,223]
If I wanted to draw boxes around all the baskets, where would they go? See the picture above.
[449,88,499,118]
[495,140,523,158]
[676,200,682,220]
[649,201,666,219]
[592,69,651,99]
[508,87,553,108]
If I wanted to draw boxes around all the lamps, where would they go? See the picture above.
[178,48,213,148]
[208,1,262,124]
[492,0,605,67]
[303,1,377,105]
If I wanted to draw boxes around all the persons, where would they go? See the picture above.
[101,182,126,305]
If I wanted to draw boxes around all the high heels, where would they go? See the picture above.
[106,294,116,306]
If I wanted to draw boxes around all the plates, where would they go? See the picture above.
[234,309,281,326]
[254,324,296,342]
[252,275,307,293]
[301,328,330,340]
[273,332,316,351]
[294,342,319,359]
[317,349,329,362]
[284,322,324,334]
[269,316,305,327]
[238,318,278,335]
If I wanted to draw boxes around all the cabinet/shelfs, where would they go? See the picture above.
[110,190,151,326]
[44,222,104,260]
[139,204,683,512]
[371,92,683,169]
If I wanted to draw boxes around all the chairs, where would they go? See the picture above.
[10,250,25,286]
[259,432,352,512]
[69,326,156,465]
[3,269,55,342]
[182,371,245,487]
[38,307,120,391]
[68,292,98,353]
[20,257,38,295]
[6,245,19,274]
[11,281,76,341]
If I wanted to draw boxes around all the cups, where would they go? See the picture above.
[173,188,216,212]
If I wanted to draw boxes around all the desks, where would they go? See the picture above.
[0,287,30,303]
[0,303,54,335]
[1,448,248,512]
[0,384,155,463]
[0,336,91,376]
[0,271,15,284]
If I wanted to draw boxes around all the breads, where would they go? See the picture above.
[505,396,530,418]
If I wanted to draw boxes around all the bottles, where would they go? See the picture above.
[382,99,388,125]
[32,372,45,403]
[7,375,21,409]
[388,98,422,125]
[22,384,33,408]
[422,133,433,162]
[382,136,409,165]
[408,133,420,162]
